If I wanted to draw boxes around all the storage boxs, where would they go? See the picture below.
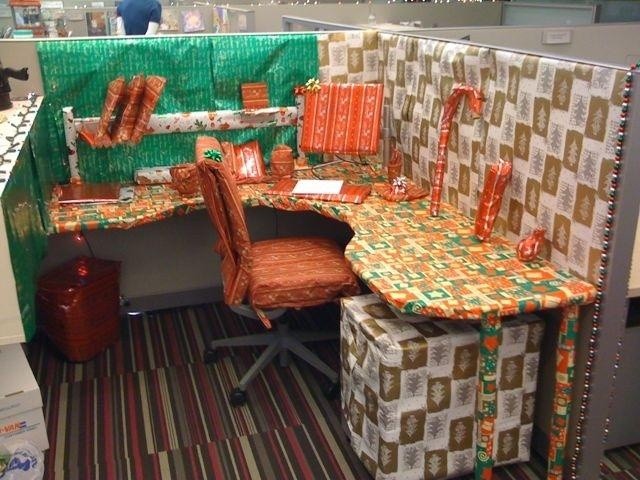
[339,293,545,480]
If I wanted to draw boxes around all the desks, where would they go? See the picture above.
[0,29,640,479]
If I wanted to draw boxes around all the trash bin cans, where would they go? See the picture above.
[38,255,122,361]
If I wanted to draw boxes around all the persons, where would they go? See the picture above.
[116,0,162,37]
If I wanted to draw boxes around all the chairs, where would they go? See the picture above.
[195,137,361,405]
